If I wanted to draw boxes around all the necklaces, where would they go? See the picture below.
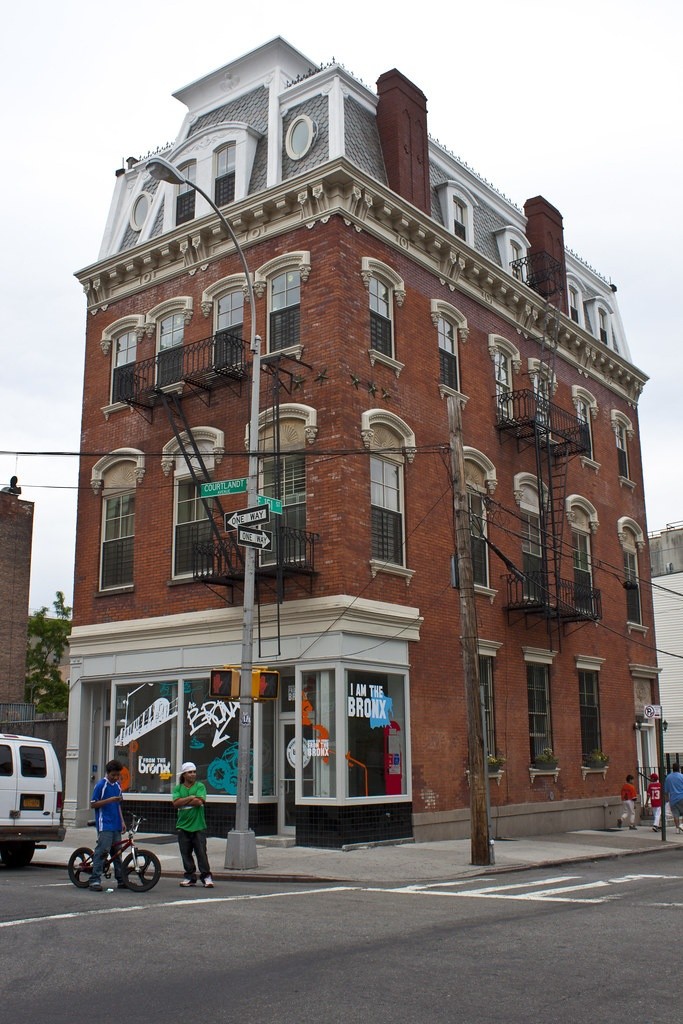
[184,783,193,788]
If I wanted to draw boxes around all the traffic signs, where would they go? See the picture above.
[224,503,270,532]
[236,524,273,553]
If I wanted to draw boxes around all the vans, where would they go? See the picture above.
[0,733,66,868]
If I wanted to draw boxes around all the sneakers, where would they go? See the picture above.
[90,882,102,891]
[204,875,215,888]
[117,882,137,889]
[180,878,197,886]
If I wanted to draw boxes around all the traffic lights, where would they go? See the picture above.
[252,670,280,700]
[208,667,240,701]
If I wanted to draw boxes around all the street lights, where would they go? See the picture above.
[143,155,262,870]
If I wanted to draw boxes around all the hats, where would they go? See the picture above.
[649,774,659,780]
[672,763,679,769]
[178,762,196,774]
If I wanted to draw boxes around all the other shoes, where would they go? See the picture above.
[658,827,662,831]
[653,826,658,832]
[617,818,623,828]
[674,828,680,833]
[679,824,683,831]
[629,825,637,830]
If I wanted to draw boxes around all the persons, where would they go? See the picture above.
[88,760,137,891]
[664,763,683,834]
[173,762,214,888]
[617,775,638,830]
[645,773,662,832]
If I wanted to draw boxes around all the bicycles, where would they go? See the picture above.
[68,811,161,893]
